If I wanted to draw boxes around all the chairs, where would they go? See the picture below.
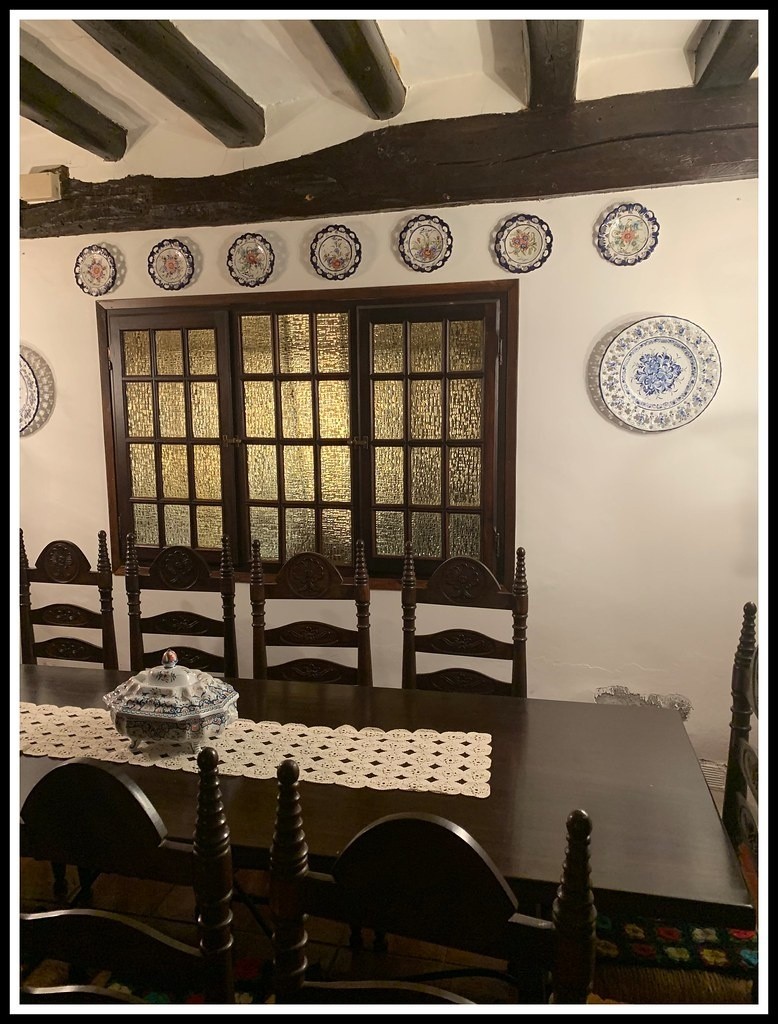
[19,747,274,1004]
[19,527,119,671]
[587,603,759,1005]
[402,542,527,696]
[271,762,596,1004]
[251,539,372,686]
[126,533,239,679]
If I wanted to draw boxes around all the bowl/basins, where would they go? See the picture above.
[101,649,239,753]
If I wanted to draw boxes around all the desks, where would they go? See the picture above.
[21,664,755,1006]
